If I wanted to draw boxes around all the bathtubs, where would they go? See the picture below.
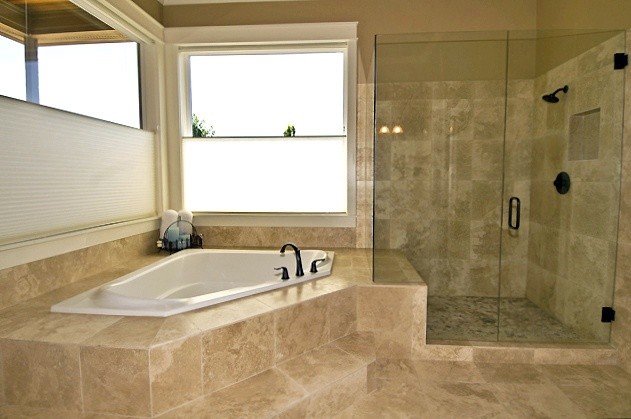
[50,249,333,316]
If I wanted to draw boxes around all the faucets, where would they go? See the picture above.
[280,243,304,276]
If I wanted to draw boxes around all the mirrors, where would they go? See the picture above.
[0,0,144,129]
